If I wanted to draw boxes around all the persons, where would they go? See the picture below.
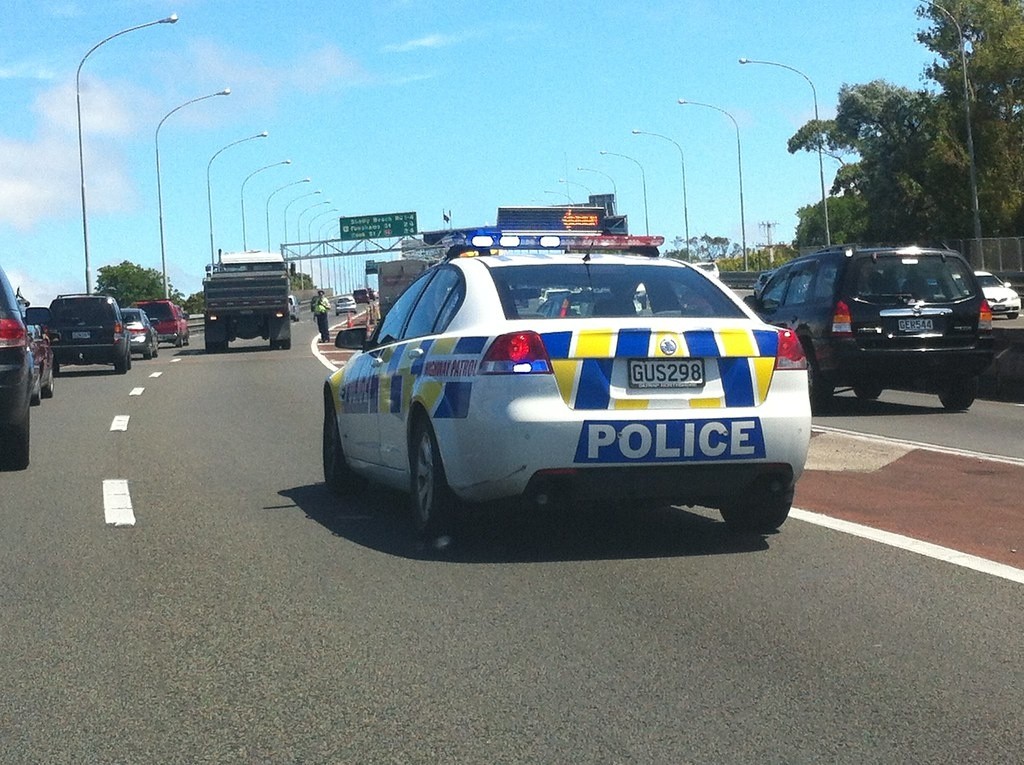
[313,289,331,343]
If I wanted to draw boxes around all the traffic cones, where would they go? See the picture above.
[365,316,372,338]
[347,313,354,328]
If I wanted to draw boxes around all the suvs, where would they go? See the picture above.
[135,301,191,347]
[536,294,618,317]
[46,294,135,375]
[745,242,993,412]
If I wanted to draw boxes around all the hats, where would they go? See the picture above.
[318,290,325,294]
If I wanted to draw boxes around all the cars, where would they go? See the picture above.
[934,271,1021,318]
[121,306,162,359]
[320,236,812,538]
[0,267,55,470]
[334,296,359,315]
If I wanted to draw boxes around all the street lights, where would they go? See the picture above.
[632,128,690,261]
[241,159,339,293]
[600,151,648,237]
[545,168,618,215]
[76,13,176,293]
[153,86,231,300]
[207,130,267,272]
[737,59,830,251]
[679,99,748,271]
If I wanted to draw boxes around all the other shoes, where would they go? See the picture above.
[319,339,329,343]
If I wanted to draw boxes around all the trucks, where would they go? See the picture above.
[202,250,296,353]
